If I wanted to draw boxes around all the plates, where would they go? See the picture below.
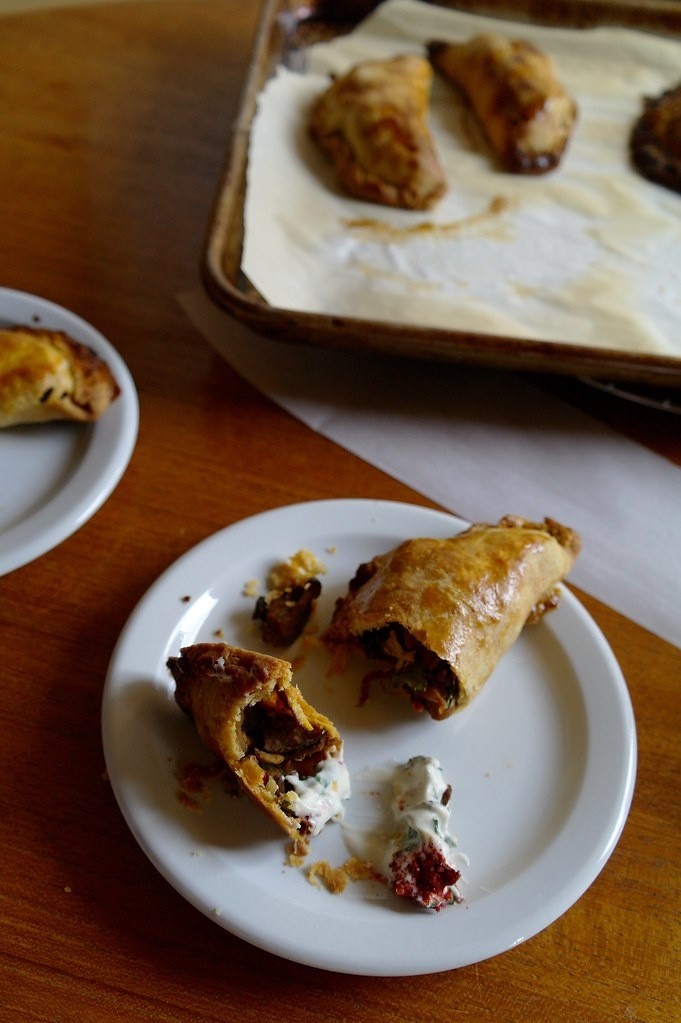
[102,499,637,976]
[200,0,681,391]
[0,285,139,576]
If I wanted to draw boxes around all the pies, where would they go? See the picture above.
[309,33,577,208]
[168,514,582,848]
[631,83,681,195]
[1,323,121,427]
